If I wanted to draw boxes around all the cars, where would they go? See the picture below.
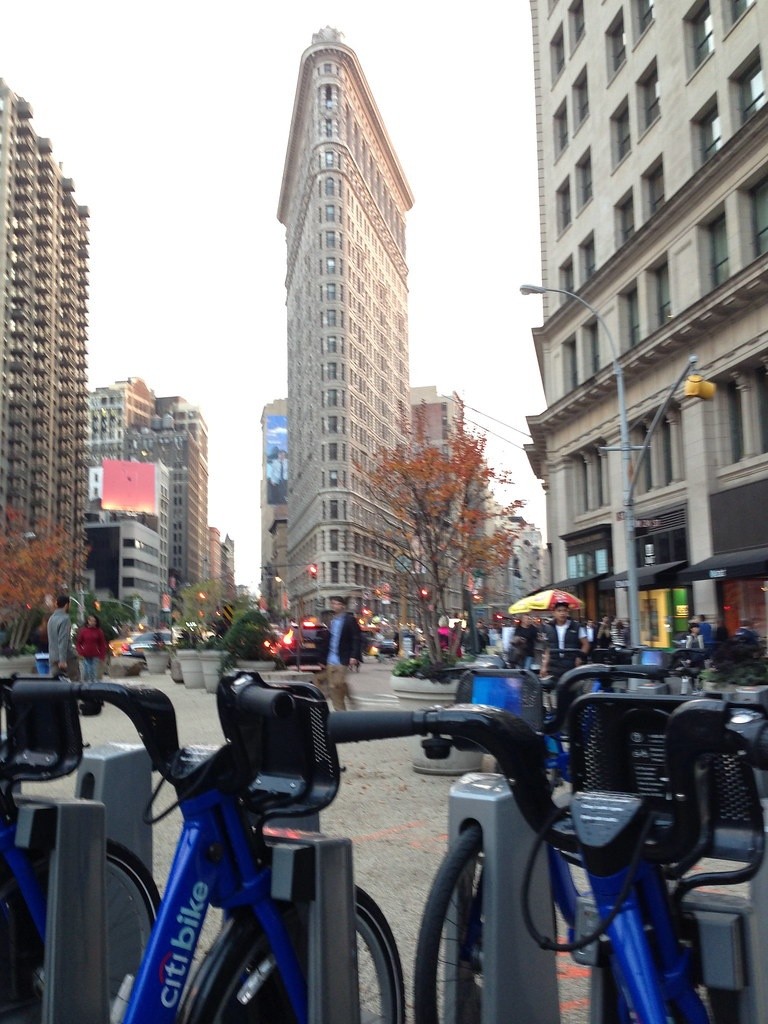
[121,619,332,672]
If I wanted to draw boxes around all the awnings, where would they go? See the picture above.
[528,572,609,595]
[677,547,768,582]
[599,560,689,590]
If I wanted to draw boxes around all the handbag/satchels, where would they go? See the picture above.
[507,644,525,665]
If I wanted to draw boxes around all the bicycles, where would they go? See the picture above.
[1,646,768,1024]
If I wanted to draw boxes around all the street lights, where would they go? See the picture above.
[521,282,640,645]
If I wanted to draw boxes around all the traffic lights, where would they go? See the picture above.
[310,564,318,579]
[683,375,718,400]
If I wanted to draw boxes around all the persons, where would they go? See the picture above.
[319,596,362,710]
[47,596,71,675]
[435,602,758,685]
[266,450,288,506]
[76,614,106,682]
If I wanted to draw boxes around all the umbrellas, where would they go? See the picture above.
[508,589,585,614]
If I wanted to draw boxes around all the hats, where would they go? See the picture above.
[553,601,569,609]
